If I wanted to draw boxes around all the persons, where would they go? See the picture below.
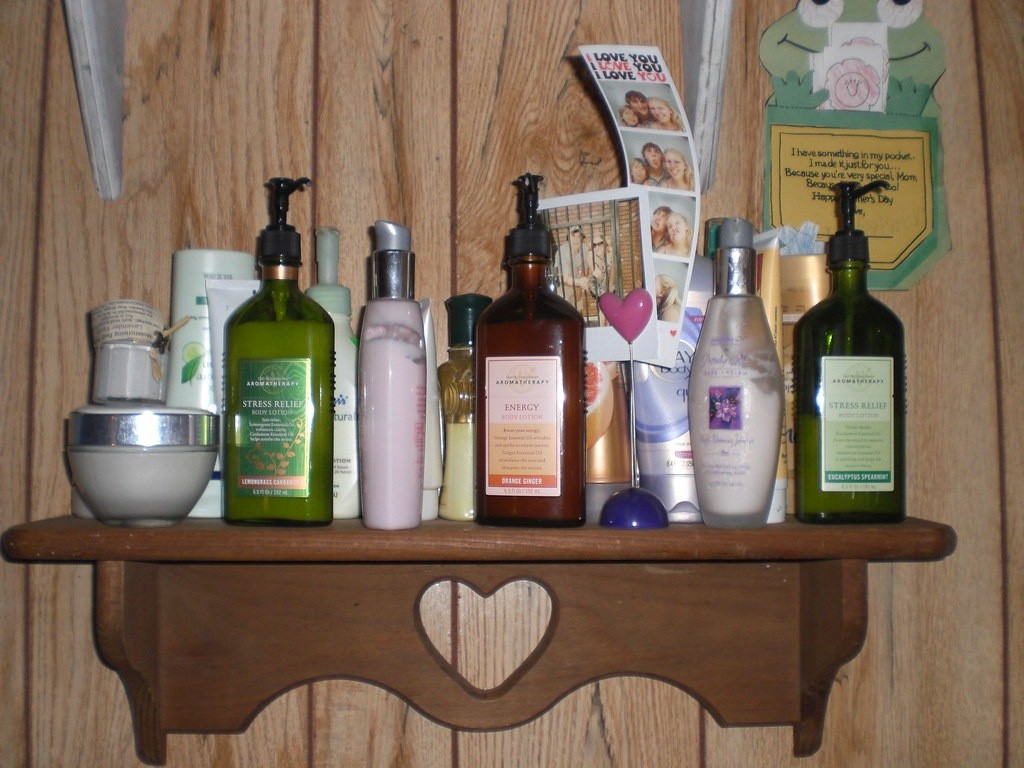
[552,222,591,313]
[646,96,686,131]
[624,90,654,129]
[586,230,617,326]
[641,143,668,186]
[618,105,644,129]
[658,211,693,256]
[655,272,680,324]
[629,157,649,183]
[658,148,696,191]
[651,206,672,253]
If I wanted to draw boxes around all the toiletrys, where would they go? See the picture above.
[578,362,641,528]
[409,295,446,525]
[203,276,259,470]
[787,171,910,524]
[633,281,728,526]
[746,221,790,522]
[220,177,339,527]
[780,255,834,513]
[294,224,366,522]
[469,172,588,528]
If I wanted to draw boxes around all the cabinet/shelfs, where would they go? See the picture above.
[2,513,959,767]
